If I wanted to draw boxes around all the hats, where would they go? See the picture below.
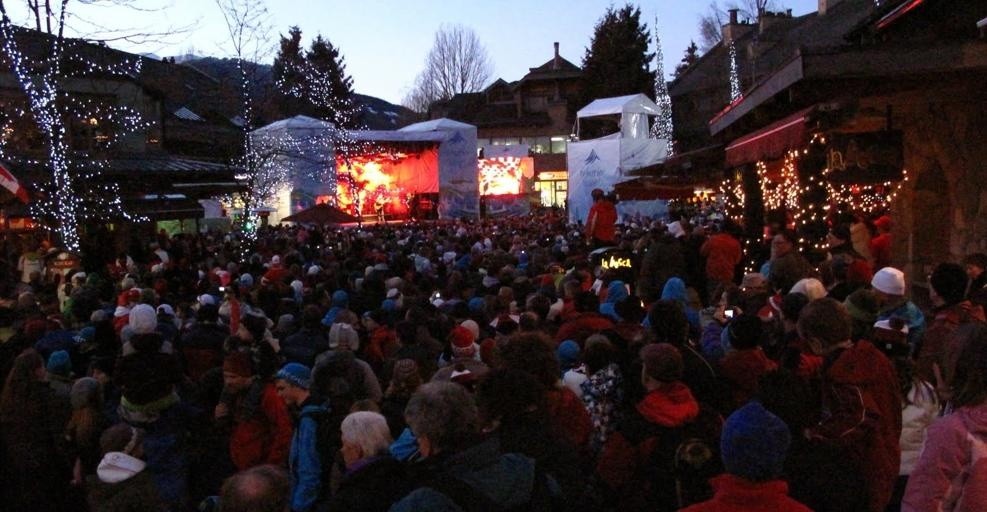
[71,221,686,348]
[221,352,251,378]
[98,422,147,461]
[727,311,761,351]
[929,260,968,305]
[789,276,830,306]
[870,265,907,298]
[390,357,418,389]
[777,293,809,323]
[739,271,771,289]
[68,377,102,413]
[45,351,73,378]
[116,349,180,411]
[871,333,909,362]
[271,362,312,393]
[717,400,792,483]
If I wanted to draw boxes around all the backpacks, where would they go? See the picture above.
[654,417,722,509]
[295,409,342,500]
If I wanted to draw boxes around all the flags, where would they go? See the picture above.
[0,163,34,205]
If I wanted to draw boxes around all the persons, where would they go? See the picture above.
[0,186,986,510]
